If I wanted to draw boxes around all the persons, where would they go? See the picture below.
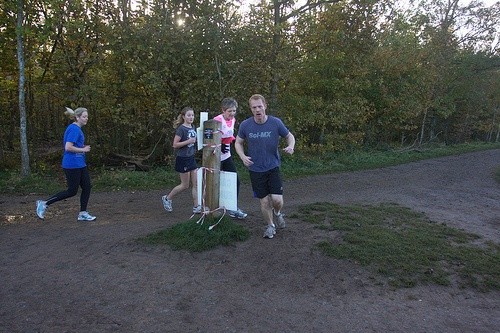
[36,107,96,221]
[213,98,247,219]
[162,107,210,213]
[235,94,295,239]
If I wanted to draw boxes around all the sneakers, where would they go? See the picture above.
[272,208,286,229]
[229,209,248,219]
[162,195,173,212]
[36,200,48,218]
[77,211,96,221]
[263,225,276,238]
[193,205,208,213]
